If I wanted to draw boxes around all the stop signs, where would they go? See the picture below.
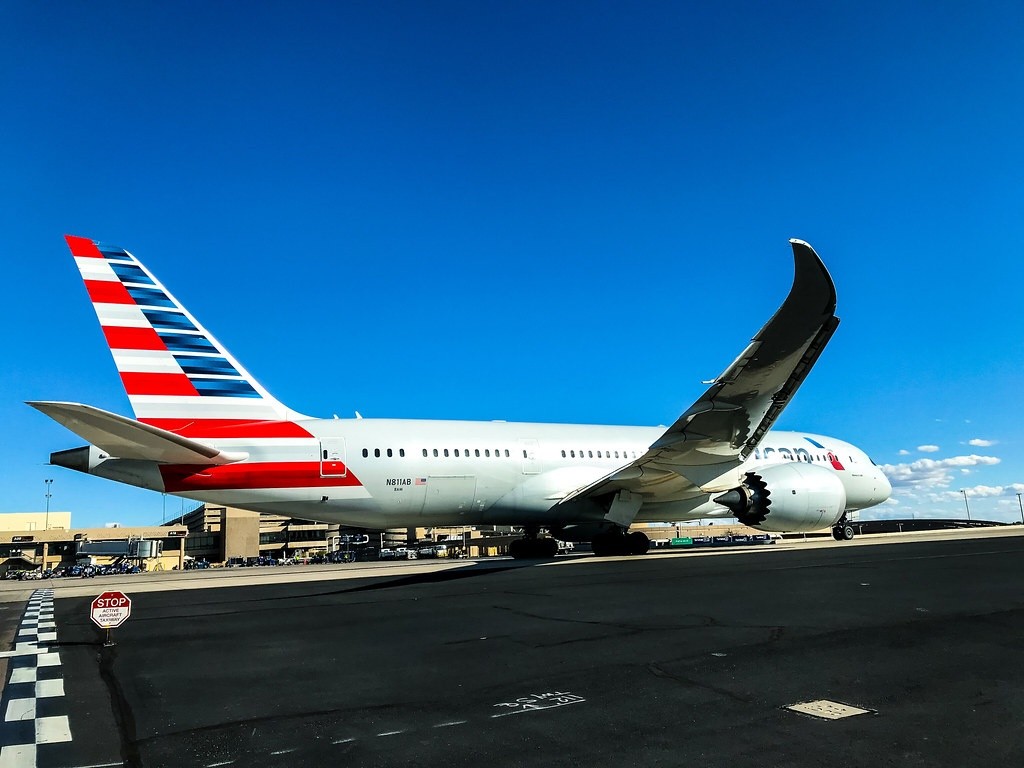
[89,590,133,628]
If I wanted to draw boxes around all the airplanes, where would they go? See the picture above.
[22,231,893,558]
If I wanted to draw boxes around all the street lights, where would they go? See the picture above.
[961,489,971,520]
[1016,493,1024,523]
[44,479,54,529]
